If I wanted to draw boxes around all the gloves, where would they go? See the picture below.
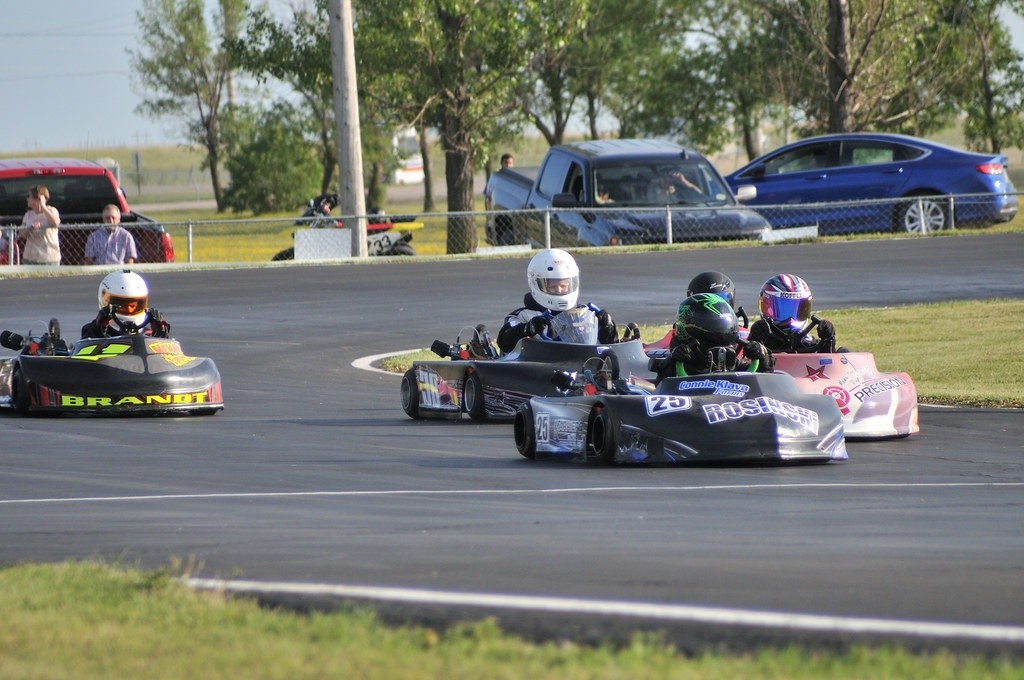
[147,308,163,328]
[743,341,770,370]
[524,316,551,337]
[96,304,122,329]
[817,320,835,341]
[596,309,614,328]
[671,344,693,362]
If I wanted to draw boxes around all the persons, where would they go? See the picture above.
[483,153,514,231]
[580,173,610,202]
[674,270,736,329]
[81,270,170,339]
[651,162,709,204]
[19,184,62,265]
[654,292,777,387]
[85,204,137,265]
[497,248,616,354]
[745,273,850,353]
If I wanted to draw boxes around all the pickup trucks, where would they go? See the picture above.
[482,138,772,250]
[0,157,176,265]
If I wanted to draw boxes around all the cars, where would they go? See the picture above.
[706,131,1020,233]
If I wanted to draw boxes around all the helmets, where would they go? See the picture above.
[98,269,149,334]
[675,293,738,374]
[759,274,813,336]
[527,249,579,311]
[687,271,735,309]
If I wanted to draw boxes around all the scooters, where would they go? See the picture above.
[271,192,417,261]
